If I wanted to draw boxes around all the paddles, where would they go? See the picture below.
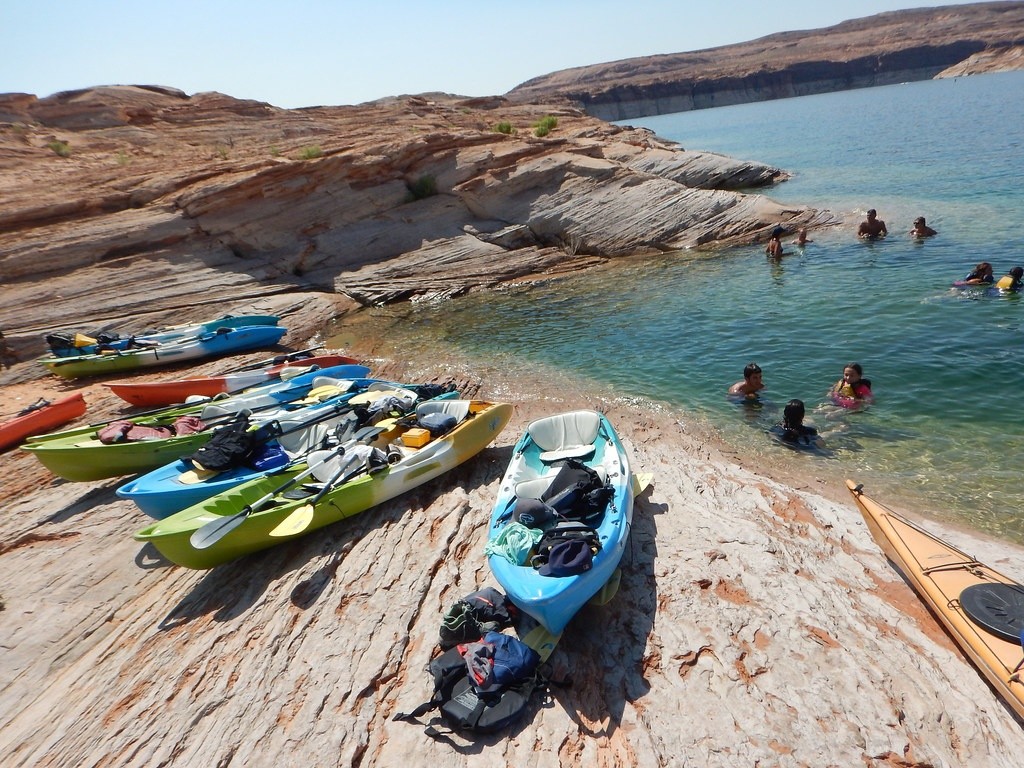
[519,472,655,665]
[182,332,356,381]
[375,411,416,435]
[177,390,382,485]
[194,385,340,436]
[188,426,387,551]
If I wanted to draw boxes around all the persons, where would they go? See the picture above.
[856,209,888,239]
[999,266,1024,287]
[837,362,873,399]
[792,229,814,245]
[778,398,817,440]
[909,216,937,235]
[966,263,994,284]
[766,226,786,256]
[729,362,765,394]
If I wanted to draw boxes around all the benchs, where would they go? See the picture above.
[415,399,472,430]
[513,465,608,530]
[525,410,601,462]
[198,405,252,431]
[311,377,354,400]
[299,451,372,491]
[364,381,418,410]
[278,365,322,381]
[274,419,329,461]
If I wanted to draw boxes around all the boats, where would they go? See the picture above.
[0,391,88,463]
[36,313,290,375]
[18,350,516,573]
[482,408,638,634]
[845,477,1024,726]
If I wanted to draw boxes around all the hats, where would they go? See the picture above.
[507,498,545,530]
[540,540,592,578]
